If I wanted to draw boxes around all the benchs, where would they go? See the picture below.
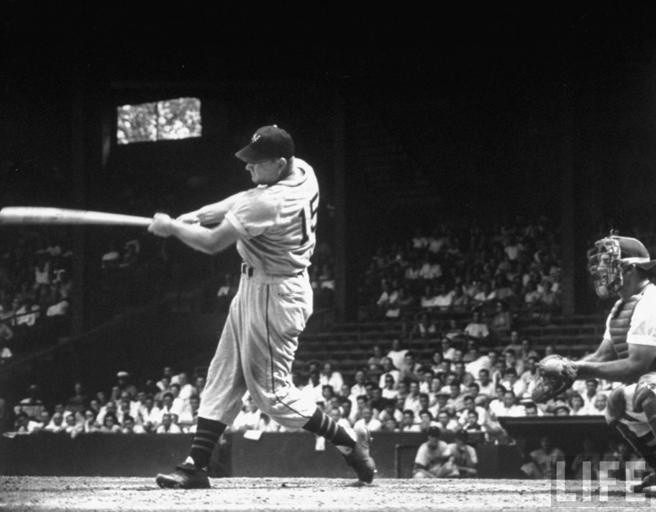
[292,305,606,379]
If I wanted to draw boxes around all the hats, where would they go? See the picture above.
[235,123,294,166]
[586,228,655,270]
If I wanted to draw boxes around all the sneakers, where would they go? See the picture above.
[155,454,211,489]
[632,474,656,496]
[336,440,376,482]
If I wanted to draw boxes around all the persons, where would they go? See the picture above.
[0,208,656,497]
[146,124,376,489]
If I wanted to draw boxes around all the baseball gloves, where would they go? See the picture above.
[531,354,577,404]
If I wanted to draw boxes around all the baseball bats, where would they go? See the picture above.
[0,206,200,228]
